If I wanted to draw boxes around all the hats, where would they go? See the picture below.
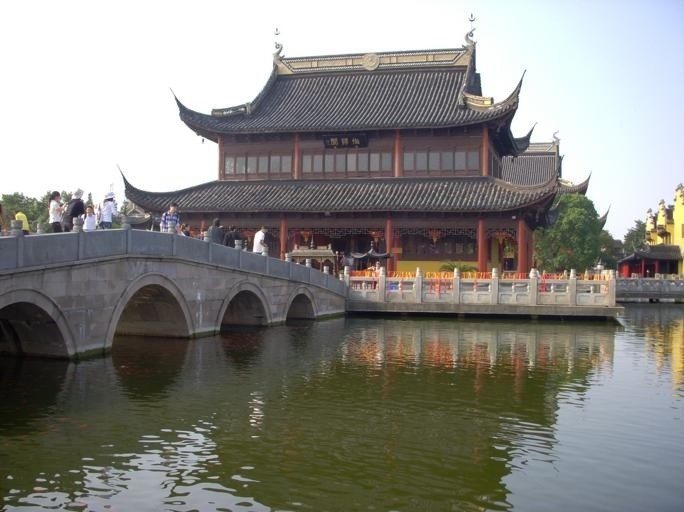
[104,192,115,200]
[71,188,84,201]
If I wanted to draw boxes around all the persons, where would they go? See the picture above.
[100,192,125,229]
[49,191,68,232]
[529,263,540,278]
[14,208,30,234]
[63,188,85,232]
[82,207,98,230]
[159,203,269,255]
[368,261,380,290]
